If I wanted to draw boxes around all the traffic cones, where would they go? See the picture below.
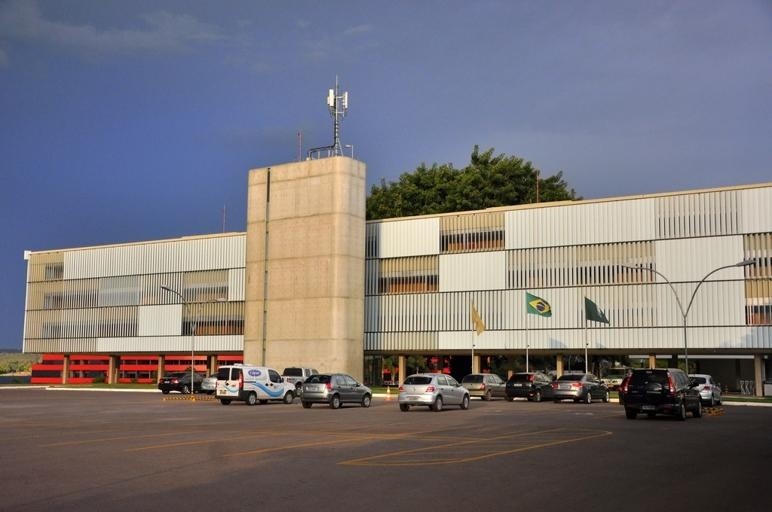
[384,385,394,402]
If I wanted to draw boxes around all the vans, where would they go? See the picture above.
[215,363,297,406]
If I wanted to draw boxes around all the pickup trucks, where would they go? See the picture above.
[280,367,319,394]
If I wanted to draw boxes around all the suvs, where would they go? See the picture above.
[687,373,723,406]
[622,367,703,421]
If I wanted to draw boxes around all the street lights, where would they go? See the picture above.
[159,284,227,395]
[620,258,757,375]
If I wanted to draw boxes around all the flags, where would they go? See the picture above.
[471,300,485,339]
[584,294,610,325]
[523,288,552,320]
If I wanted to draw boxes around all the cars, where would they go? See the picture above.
[299,374,372,410]
[462,373,506,400]
[158,371,202,394]
[398,373,470,411]
[506,371,555,402]
[200,373,217,394]
[618,377,629,405]
[552,373,610,404]
